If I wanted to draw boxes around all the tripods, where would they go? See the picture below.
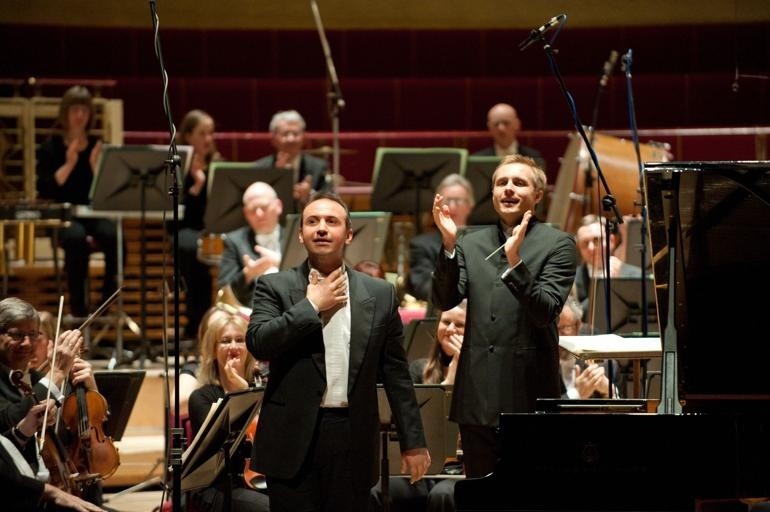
[85,214,159,372]
[107,170,169,367]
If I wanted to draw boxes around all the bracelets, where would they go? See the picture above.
[14,425,28,441]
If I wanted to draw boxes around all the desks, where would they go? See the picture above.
[74,205,184,369]
[558,334,663,399]
[197,249,224,265]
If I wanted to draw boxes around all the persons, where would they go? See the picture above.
[555,294,618,399]
[217,181,286,310]
[189,315,269,510]
[432,156,577,480]
[684,180,770,498]
[169,300,244,420]
[247,191,429,510]
[475,103,544,224]
[35,85,126,326]
[30,311,101,505]
[406,175,476,301]
[258,112,329,213]
[566,215,655,323]
[1,296,107,512]
[410,305,467,386]
[167,110,224,340]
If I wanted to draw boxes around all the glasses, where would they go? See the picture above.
[275,129,302,137]
[441,197,469,207]
[2,330,43,342]
[216,337,246,347]
[555,325,577,337]
[577,237,602,249]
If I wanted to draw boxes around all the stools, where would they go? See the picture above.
[52,234,99,358]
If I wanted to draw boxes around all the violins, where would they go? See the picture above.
[8,366,83,502]
[61,327,119,484]
[242,362,270,493]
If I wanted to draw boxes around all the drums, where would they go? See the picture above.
[545,123,679,266]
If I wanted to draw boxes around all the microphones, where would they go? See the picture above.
[600,50,618,87]
[516,12,567,54]
[619,49,633,72]
